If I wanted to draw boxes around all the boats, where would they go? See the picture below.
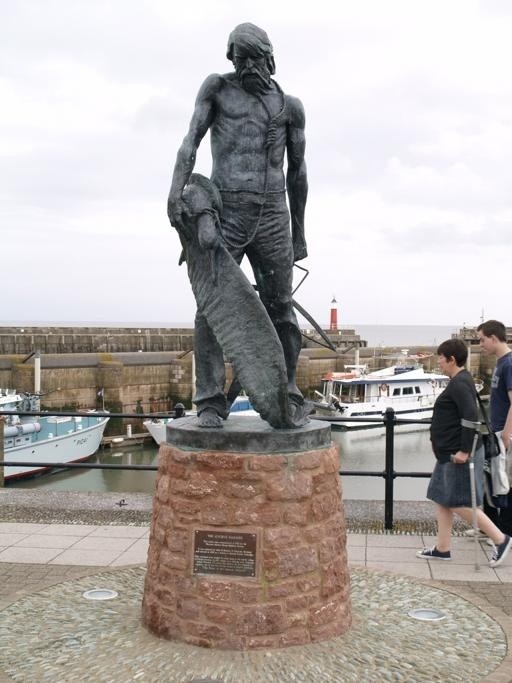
[0,384,112,484]
[142,389,262,448]
[307,359,487,430]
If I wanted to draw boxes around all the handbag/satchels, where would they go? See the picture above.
[481,429,501,460]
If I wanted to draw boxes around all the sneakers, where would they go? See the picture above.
[416,545,452,561]
[464,527,489,539]
[488,532,512,569]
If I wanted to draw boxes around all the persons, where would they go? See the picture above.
[167,18,309,428]
[462,319,512,547]
[417,338,511,567]
